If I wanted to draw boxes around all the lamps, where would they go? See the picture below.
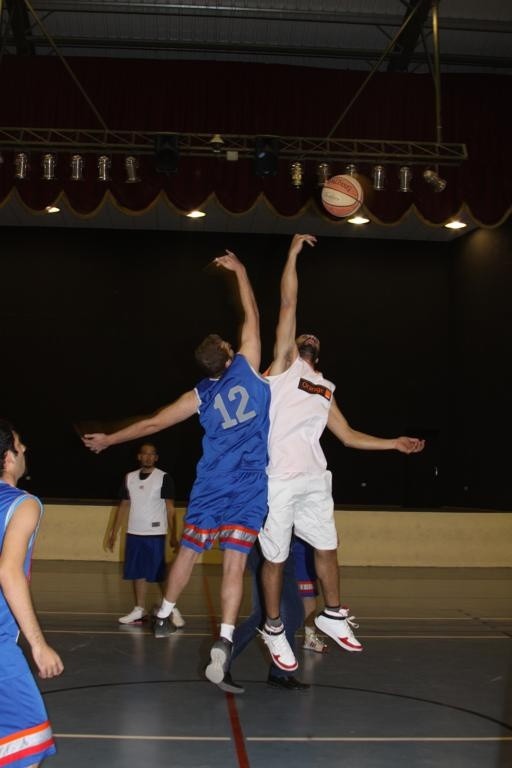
[13,145,140,187]
[290,158,450,195]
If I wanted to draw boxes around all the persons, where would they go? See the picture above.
[294,539,328,656]
[203,540,312,692]
[109,443,184,626]
[1,425,64,767]
[260,233,424,671]
[78,248,271,682]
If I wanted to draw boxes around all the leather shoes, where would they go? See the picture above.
[269,672,310,692]
[207,675,244,695]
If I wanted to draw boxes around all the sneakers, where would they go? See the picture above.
[119,609,184,639]
[204,636,234,684]
[260,623,298,671]
[302,633,329,654]
[312,606,364,653]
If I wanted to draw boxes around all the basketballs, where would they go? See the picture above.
[322,175,362,218]
